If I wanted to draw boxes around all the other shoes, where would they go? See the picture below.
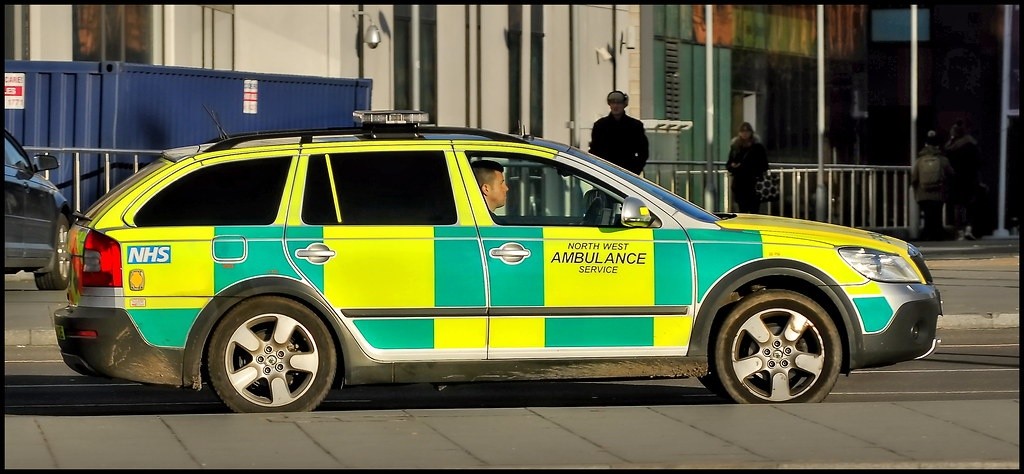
[965,227,976,241]
[956,230,964,242]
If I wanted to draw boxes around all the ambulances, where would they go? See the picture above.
[55,110,945,409]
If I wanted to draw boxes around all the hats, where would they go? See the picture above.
[926,130,940,146]
[951,120,968,137]
[607,91,628,106]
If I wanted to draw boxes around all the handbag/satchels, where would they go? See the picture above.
[754,175,780,203]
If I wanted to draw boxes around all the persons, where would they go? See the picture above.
[588,91,649,176]
[470,160,509,213]
[726,123,768,214]
[911,119,983,241]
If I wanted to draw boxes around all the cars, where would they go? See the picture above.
[1,128,77,291]
